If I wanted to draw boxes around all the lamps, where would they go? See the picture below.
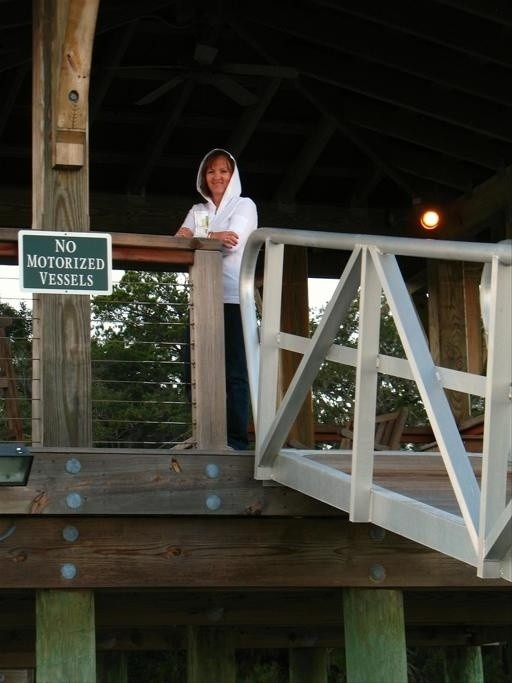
[0,446,34,486]
[415,191,448,236]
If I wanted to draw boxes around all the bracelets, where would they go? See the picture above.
[208,230,213,239]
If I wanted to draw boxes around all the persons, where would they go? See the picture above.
[170,146,263,448]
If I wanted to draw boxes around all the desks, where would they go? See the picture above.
[419,435,483,453]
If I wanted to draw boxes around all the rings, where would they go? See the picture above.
[224,238,228,241]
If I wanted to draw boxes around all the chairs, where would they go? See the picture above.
[287,406,409,451]
